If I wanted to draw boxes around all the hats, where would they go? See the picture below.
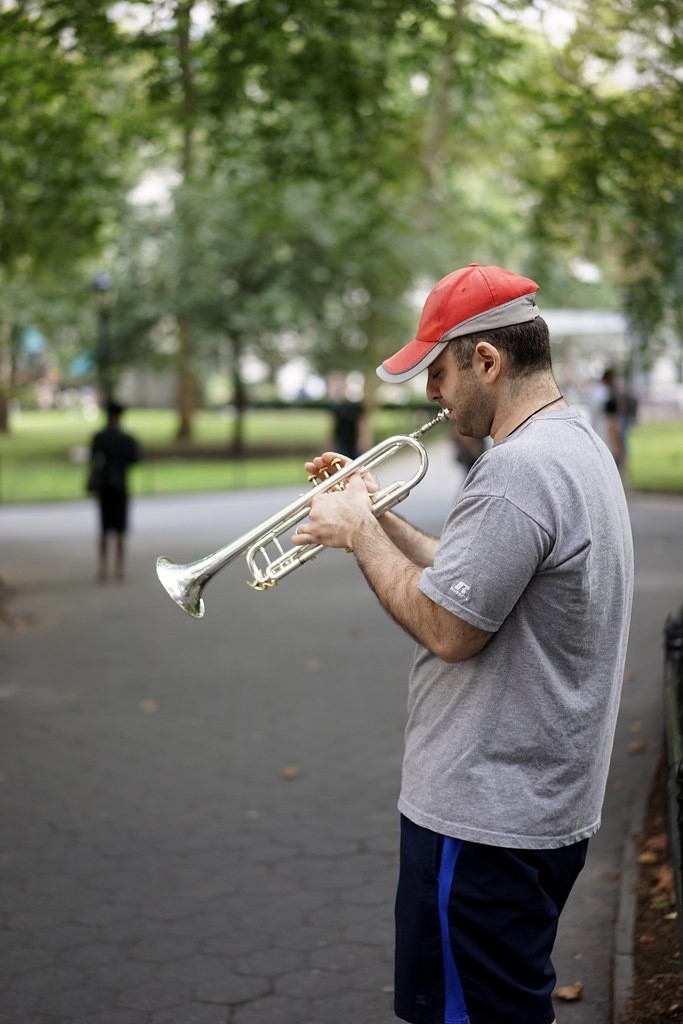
[374,263,540,386]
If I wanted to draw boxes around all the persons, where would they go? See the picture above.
[86,403,142,584]
[327,386,368,461]
[292,262,635,1024]
[592,370,638,464]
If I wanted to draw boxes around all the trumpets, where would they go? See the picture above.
[155,408,446,619]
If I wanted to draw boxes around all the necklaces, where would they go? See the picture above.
[505,394,563,438]
